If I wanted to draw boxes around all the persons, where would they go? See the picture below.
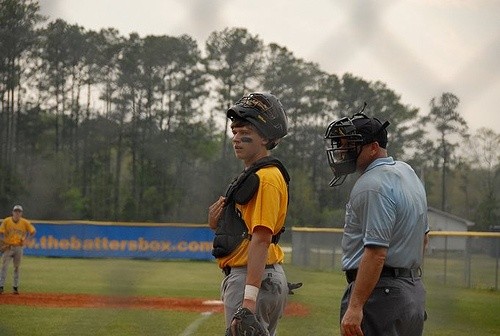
[324,113,430,336]
[0,205,35,295]
[208,92,291,336]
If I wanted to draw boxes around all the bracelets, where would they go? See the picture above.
[245,284,259,301]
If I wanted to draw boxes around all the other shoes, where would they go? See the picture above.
[13,287,19,294]
[0,289,5,294]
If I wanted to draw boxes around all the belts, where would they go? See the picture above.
[346,266,419,284]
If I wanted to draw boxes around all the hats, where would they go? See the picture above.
[13,205,23,212]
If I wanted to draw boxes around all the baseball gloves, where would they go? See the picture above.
[226,307,271,336]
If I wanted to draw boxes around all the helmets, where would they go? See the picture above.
[325,113,388,145]
[226,92,288,140]
